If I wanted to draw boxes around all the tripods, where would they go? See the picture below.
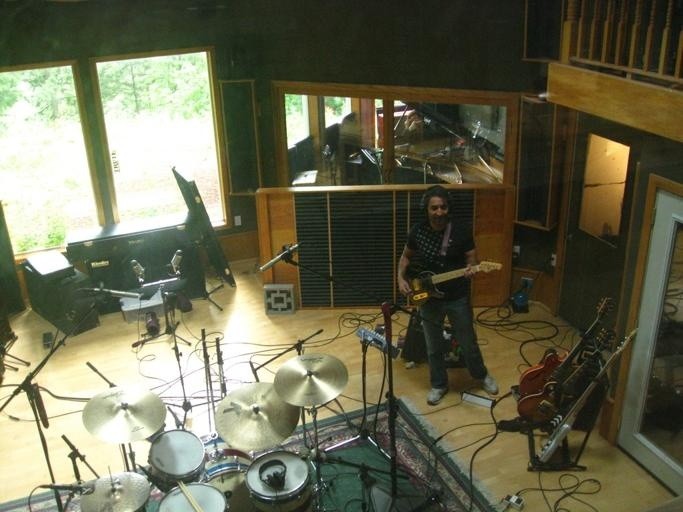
[25,252,445,511]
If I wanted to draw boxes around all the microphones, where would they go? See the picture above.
[258,241,305,273]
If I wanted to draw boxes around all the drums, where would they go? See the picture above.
[199,435,256,512]
[147,429,205,493]
[157,483,229,512]
[245,450,315,511]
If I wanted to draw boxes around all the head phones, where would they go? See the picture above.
[419,187,456,212]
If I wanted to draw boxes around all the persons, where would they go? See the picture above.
[397,186,499,405]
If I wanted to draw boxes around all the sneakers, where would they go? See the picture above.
[427,386,449,404]
[479,373,498,394]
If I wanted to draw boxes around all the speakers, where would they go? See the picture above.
[22,260,99,336]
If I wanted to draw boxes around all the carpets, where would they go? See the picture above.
[1,383,504,512]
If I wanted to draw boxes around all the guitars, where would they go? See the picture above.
[517,297,615,396]
[406,261,503,305]
[539,326,641,463]
[518,328,615,423]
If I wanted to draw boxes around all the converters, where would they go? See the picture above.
[502,494,525,512]
[513,291,529,313]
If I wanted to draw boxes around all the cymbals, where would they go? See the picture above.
[80,471,150,511]
[82,386,167,442]
[214,382,300,449]
[275,353,348,406]
